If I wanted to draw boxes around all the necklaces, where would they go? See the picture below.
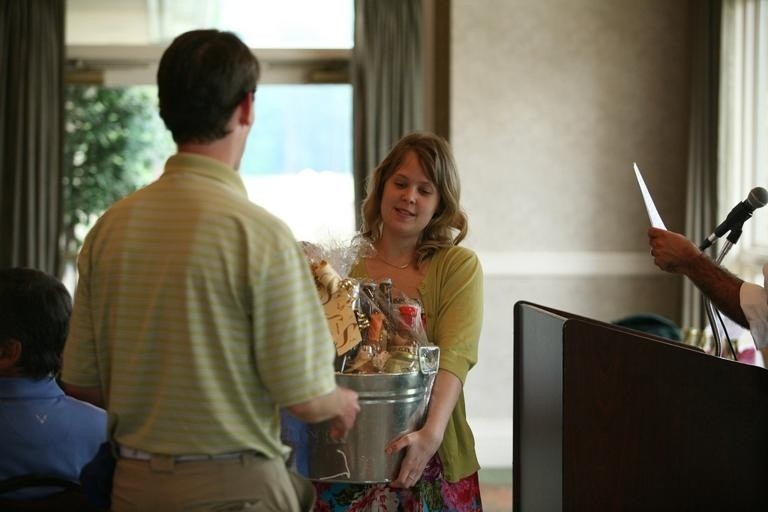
[371,249,417,271]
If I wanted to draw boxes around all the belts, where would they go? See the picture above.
[120,446,260,463]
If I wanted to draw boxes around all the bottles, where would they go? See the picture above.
[336,278,426,374]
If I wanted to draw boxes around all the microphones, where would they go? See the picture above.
[698,185,768,253]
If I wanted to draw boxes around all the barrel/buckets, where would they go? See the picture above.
[283,343,442,486]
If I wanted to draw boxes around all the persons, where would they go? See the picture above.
[314,133,488,511]
[629,223,768,377]
[57,29,361,512]
[0,266,116,511]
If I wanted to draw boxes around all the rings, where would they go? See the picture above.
[410,473,415,480]
[650,247,654,256]
